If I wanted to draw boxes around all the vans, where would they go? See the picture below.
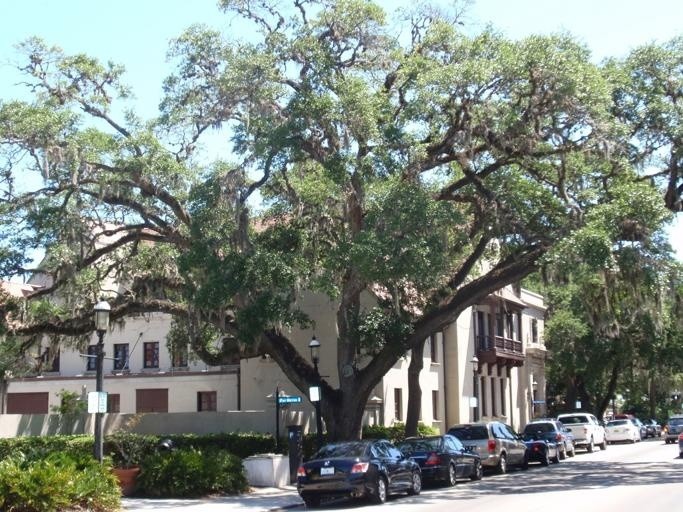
[555,412,606,453]
[448,419,531,474]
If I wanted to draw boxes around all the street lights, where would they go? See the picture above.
[469,353,481,422]
[79,300,123,464]
[532,380,539,420]
[307,335,329,450]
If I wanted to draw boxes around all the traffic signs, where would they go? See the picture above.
[276,396,303,404]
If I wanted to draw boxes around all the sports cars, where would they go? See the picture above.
[512,430,561,466]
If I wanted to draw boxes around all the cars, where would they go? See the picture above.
[525,420,577,459]
[394,435,484,484]
[296,437,424,510]
[606,407,683,457]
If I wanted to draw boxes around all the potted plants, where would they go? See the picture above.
[113,435,140,497]
